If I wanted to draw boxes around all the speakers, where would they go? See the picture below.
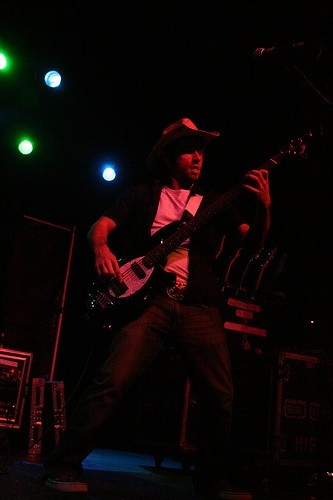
[265,351,333,468]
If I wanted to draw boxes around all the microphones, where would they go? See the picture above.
[254,41,325,64]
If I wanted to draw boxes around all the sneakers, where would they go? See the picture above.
[190,469,250,500]
[38,455,87,493]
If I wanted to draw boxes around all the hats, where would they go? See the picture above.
[146,116,221,165]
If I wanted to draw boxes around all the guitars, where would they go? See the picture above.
[82,126,325,332]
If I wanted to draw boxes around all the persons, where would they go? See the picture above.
[40,117,271,493]
